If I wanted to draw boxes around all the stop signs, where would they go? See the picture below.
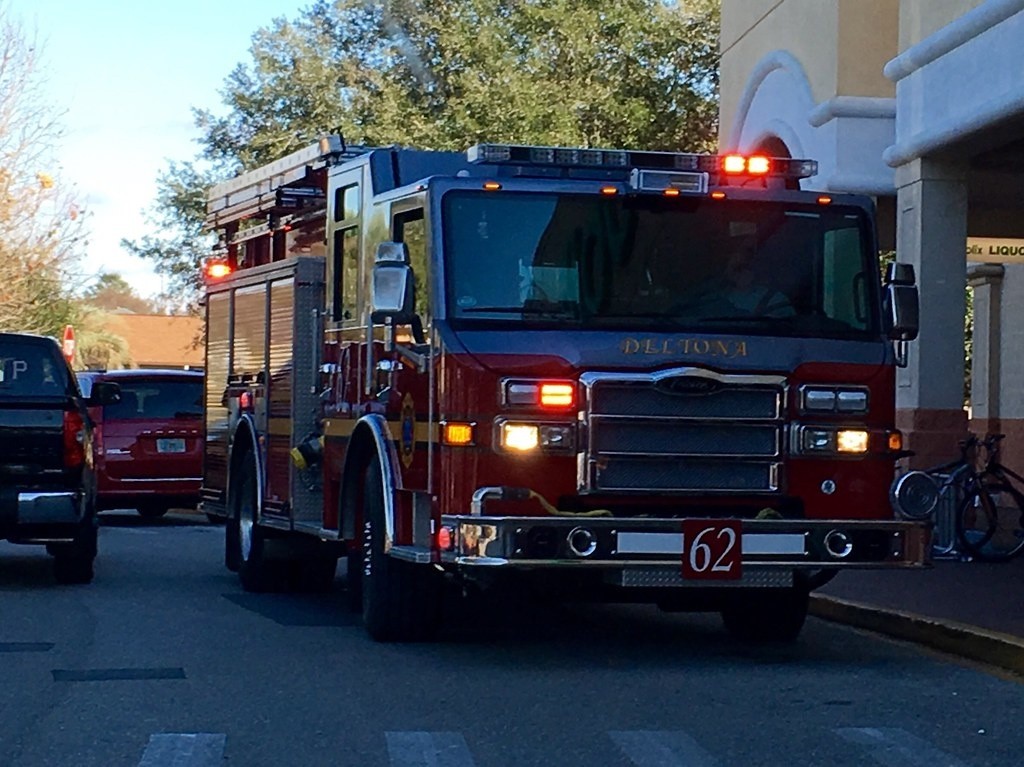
[61,324,78,361]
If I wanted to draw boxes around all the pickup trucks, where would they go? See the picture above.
[0,334,123,584]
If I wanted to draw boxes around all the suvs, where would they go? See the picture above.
[71,370,205,522]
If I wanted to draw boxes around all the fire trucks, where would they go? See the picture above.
[199,126,918,638]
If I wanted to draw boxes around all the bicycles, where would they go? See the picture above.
[893,427,1024,563]
